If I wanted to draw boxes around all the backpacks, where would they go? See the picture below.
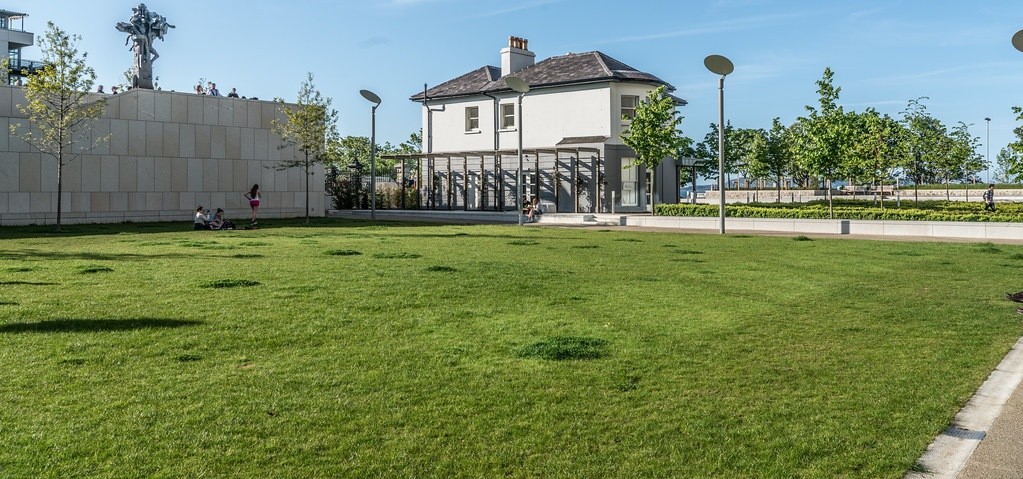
[983,191,990,199]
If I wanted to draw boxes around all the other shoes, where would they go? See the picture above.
[1017,308,1023,312]
[1006,291,1023,302]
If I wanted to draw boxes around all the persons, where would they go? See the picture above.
[244,184,262,226]
[195,205,235,230]
[523,198,543,223]
[983,184,995,212]
[96,85,119,95]
[408,175,415,188]
[197,82,258,100]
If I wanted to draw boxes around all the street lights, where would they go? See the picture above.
[504,76,529,226]
[703,54,735,234]
[360,89,381,220]
[984,117,991,187]
